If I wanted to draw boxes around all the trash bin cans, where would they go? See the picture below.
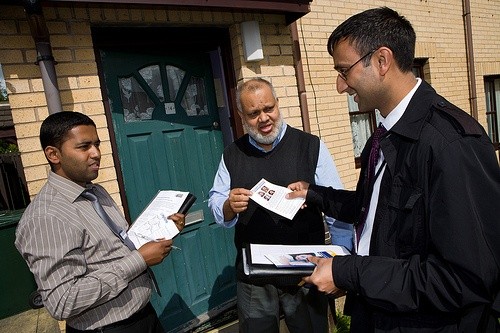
[0,214,45,320]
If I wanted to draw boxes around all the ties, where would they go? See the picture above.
[356,123,387,249]
[80,187,162,298]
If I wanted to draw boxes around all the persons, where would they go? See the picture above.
[286,5,500,333]
[210,79,354,333]
[14,110,186,333]
[278,252,316,265]
[258,185,275,201]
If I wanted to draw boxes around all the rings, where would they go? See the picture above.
[178,221,183,225]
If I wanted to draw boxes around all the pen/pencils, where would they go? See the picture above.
[143,234,181,251]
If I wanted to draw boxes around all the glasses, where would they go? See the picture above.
[334,46,379,81]
